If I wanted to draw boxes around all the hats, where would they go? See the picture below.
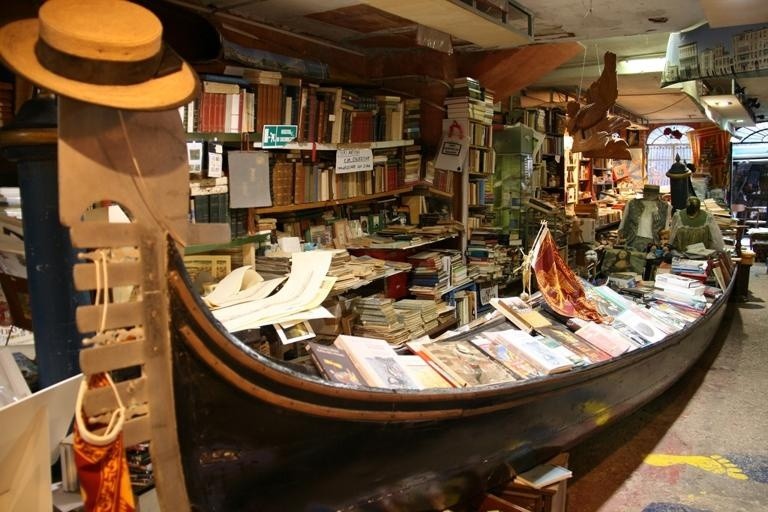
[637,185,665,197]
[0,0,204,114]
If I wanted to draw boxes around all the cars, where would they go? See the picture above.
[181,424,303,480]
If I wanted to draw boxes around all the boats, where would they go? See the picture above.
[134,218,743,511]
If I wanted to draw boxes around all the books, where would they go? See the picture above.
[178,65,736,392]
[515,461,575,493]
